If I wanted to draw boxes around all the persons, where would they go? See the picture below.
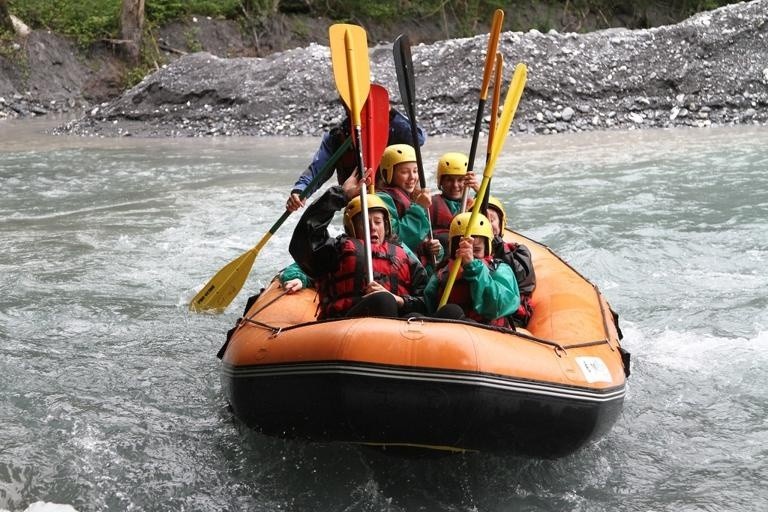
[423,212,522,328]
[374,142,445,275]
[289,167,429,320]
[429,153,481,244]
[279,193,422,295]
[465,195,536,328]
[286,95,425,212]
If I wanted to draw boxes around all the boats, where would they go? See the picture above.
[216,211,627,453]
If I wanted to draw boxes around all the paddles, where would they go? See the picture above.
[455,8,504,214]
[432,62,527,312]
[190,132,350,312]
[348,83,391,192]
[391,34,425,194]
[328,21,373,284]
[484,52,504,211]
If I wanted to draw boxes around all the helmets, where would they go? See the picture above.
[343,194,393,243]
[448,214,492,258]
[436,151,470,189]
[469,196,507,238]
[380,144,418,184]
